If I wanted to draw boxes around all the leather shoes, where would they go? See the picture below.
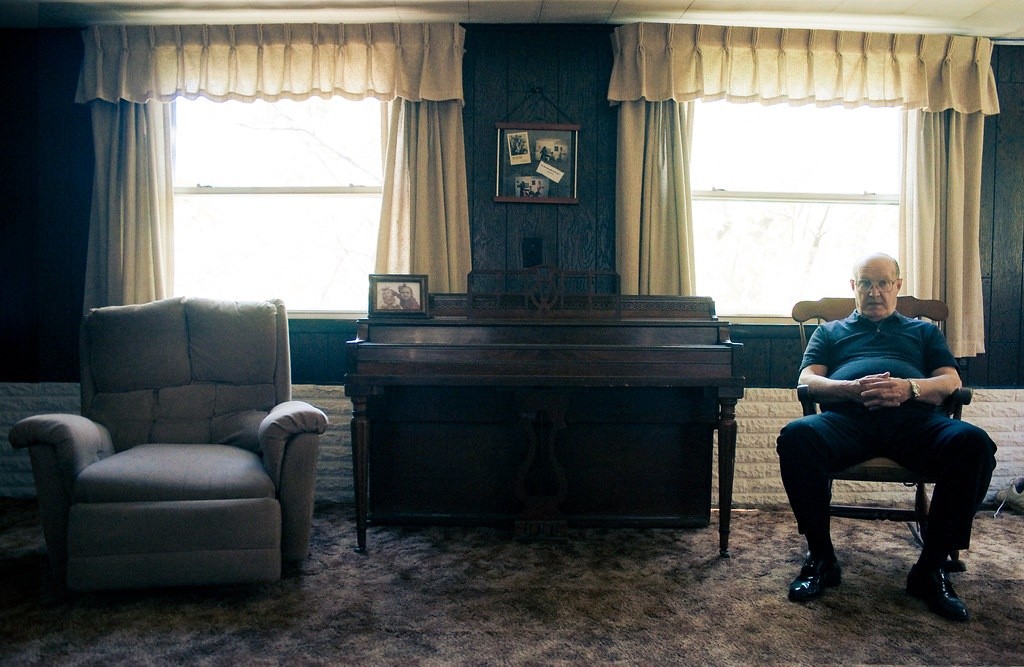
[787,557,841,600]
[906,564,969,620]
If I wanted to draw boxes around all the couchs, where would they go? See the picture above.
[8,295,330,608]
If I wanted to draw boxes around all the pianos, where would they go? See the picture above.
[344,269,745,557]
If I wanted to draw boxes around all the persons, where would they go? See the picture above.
[775,256,998,623]
[378,283,420,310]
[509,134,568,198]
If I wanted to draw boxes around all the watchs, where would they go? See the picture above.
[907,378,921,400]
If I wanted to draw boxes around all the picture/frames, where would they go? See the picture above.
[367,273,430,320]
[493,121,583,205]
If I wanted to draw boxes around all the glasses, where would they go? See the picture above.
[854,279,897,292]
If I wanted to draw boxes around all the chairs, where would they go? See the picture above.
[790,294,981,573]
